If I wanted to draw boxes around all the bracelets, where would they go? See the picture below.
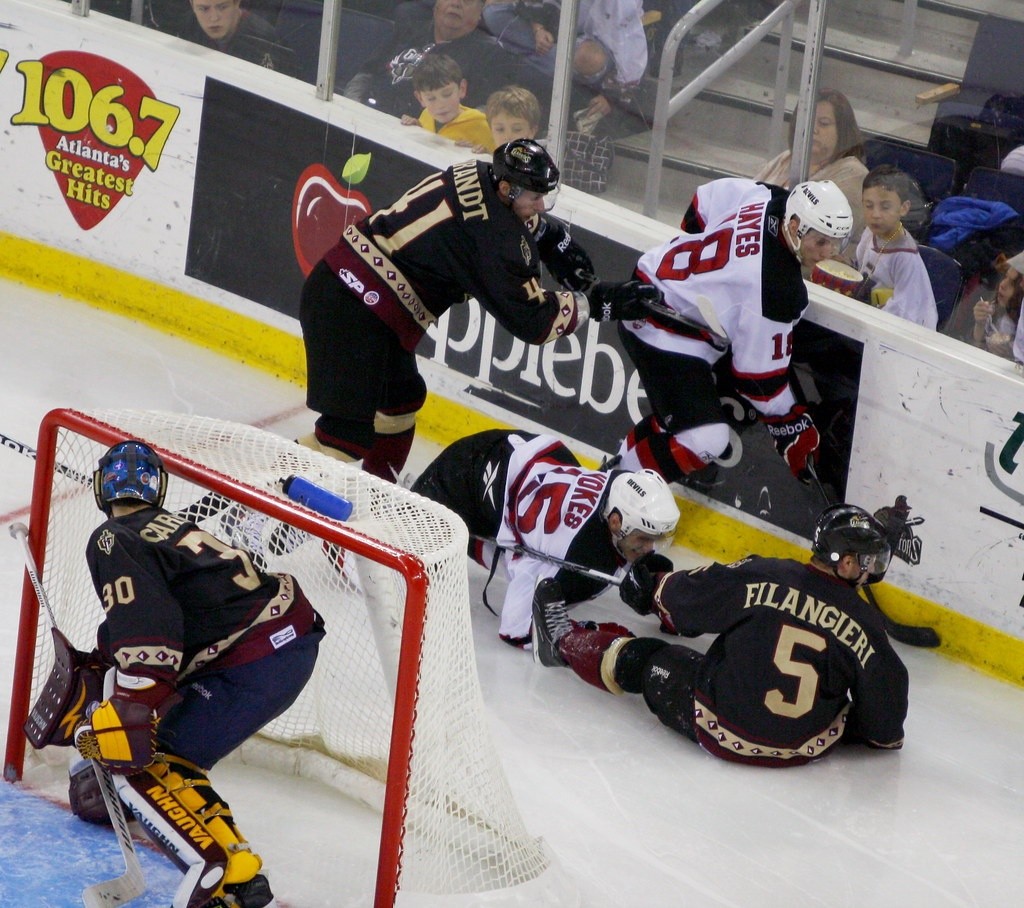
[535,25,544,34]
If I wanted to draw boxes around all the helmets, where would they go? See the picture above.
[492,137,560,193]
[92,441,168,508]
[810,503,890,584]
[782,180,853,268]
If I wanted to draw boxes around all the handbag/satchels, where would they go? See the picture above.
[541,128,614,196]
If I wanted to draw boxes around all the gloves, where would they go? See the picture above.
[618,550,674,617]
[760,405,820,486]
[589,280,664,323]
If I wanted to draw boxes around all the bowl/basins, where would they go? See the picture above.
[811,259,863,296]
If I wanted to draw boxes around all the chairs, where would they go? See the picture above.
[867,13,1023,330]
[265,1,692,124]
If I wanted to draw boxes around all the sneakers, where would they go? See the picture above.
[531,573,573,669]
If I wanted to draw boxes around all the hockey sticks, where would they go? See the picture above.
[385,458,623,587]
[804,459,942,647]
[574,266,821,410]
[9,521,148,908]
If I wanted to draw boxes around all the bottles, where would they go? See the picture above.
[279,474,353,522]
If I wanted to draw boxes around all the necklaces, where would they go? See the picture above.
[855,222,902,300]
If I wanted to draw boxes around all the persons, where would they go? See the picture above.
[531,504,909,768]
[756,90,1024,368]
[410,429,704,668]
[299,139,664,485]
[25,441,327,908]
[344,0,693,197]
[175,0,281,73]
[617,178,853,485]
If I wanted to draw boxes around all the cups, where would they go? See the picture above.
[987,337,1010,359]
